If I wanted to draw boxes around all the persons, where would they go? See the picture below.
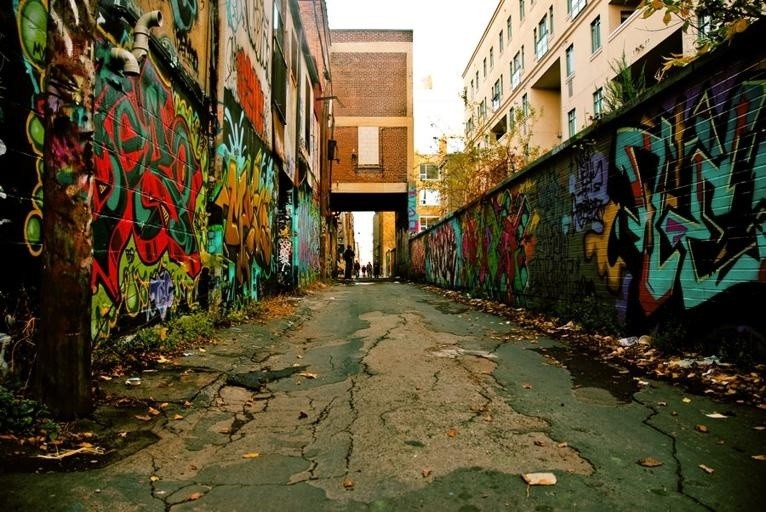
[362,265,367,277]
[367,262,372,278]
[373,260,380,279]
[354,261,360,277]
[342,245,355,278]
